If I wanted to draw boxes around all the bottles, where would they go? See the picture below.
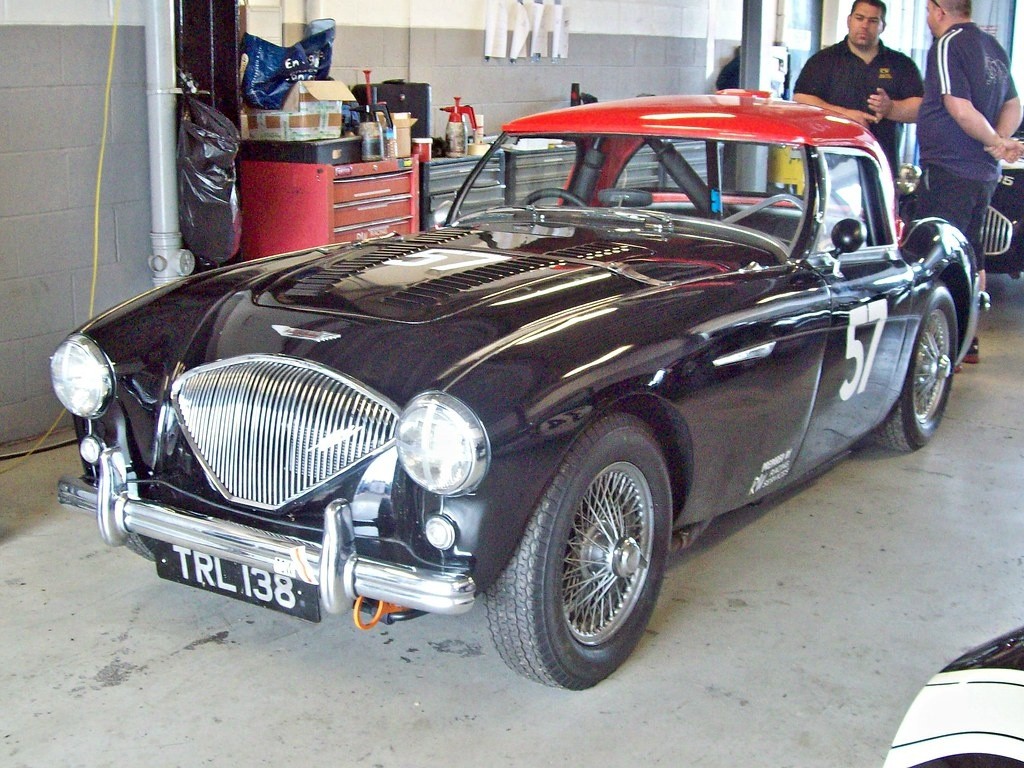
[382,117,397,161]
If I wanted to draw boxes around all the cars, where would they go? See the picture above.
[48,88,993,692]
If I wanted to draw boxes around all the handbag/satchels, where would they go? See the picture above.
[239,18,336,110]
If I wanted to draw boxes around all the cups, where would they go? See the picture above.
[412,138,432,163]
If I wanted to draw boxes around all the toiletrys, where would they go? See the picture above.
[471,113,484,143]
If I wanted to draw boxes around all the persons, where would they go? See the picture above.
[916,0,1024,374]
[716,41,792,194]
[792,0,925,178]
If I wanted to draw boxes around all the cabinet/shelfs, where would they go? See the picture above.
[237,154,420,261]
[501,139,724,205]
[420,151,506,230]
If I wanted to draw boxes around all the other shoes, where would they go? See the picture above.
[962,336,979,364]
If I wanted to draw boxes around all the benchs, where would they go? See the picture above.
[637,203,842,250]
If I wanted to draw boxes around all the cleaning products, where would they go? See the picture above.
[439,96,478,158]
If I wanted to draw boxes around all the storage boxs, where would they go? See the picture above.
[240,136,362,165]
[241,80,357,142]
[377,111,418,157]
